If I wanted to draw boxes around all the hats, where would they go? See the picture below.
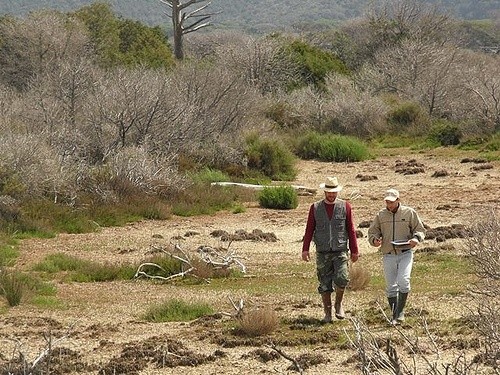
[319,177,343,193]
[384,189,399,202]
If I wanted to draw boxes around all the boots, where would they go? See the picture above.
[333,283,346,319]
[387,296,397,324]
[396,289,408,321]
[320,292,332,322]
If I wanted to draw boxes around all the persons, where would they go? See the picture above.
[301,177,359,324]
[368,189,426,325]
